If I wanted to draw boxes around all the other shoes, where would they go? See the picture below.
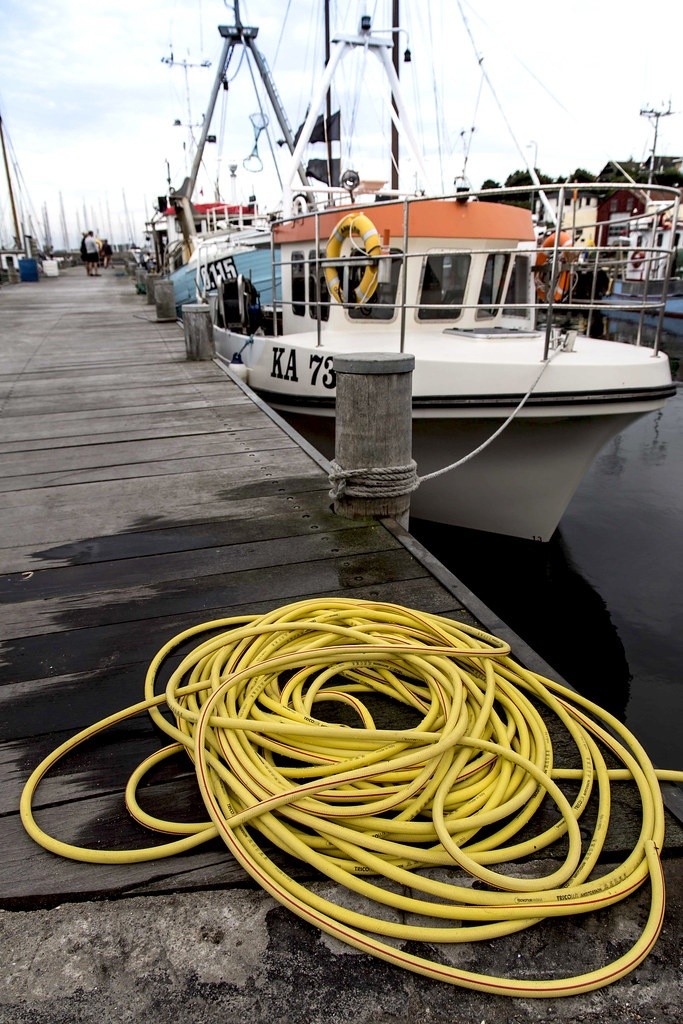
[90,273,102,277]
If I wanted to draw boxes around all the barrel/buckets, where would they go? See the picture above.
[43,260,58,277]
[20,258,37,282]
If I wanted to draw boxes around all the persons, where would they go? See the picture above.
[141,251,157,272]
[80,231,113,277]
[576,252,609,339]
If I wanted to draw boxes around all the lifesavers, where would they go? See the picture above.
[321,212,381,310]
[631,251,646,268]
[534,231,576,302]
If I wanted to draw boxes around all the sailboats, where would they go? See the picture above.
[134,0,676,554]
